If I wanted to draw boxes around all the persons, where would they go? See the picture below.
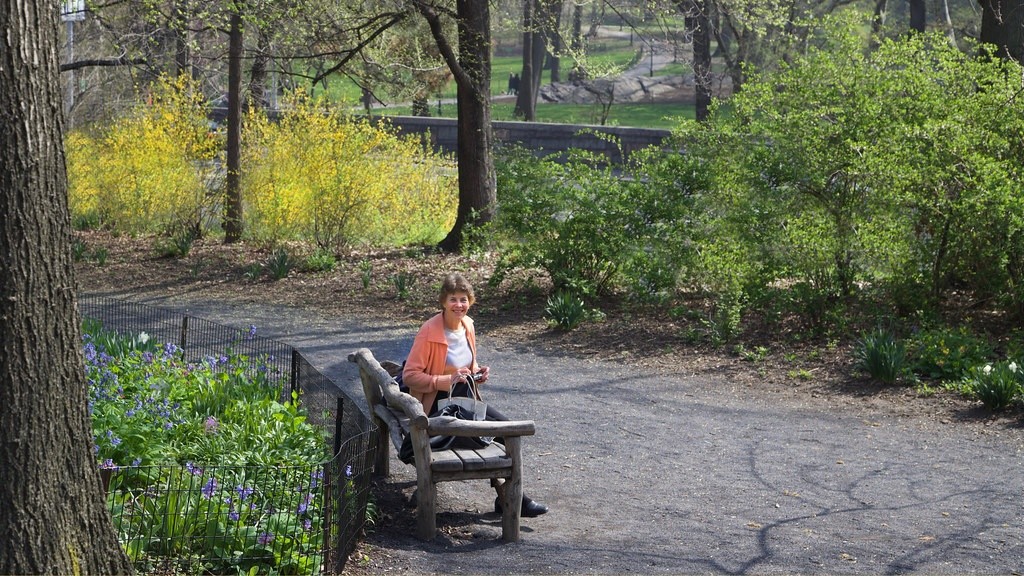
[402,273,548,518]
[507,72,520,96]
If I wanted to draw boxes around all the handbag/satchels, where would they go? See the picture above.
[437,373,488,421]
[397,404,495,465]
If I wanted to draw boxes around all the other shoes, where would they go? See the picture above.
[495,497,537,517]
[522,500,549,515]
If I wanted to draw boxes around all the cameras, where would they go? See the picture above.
[466,371,482,381]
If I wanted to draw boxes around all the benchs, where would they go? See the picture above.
[347,348,535,540]
